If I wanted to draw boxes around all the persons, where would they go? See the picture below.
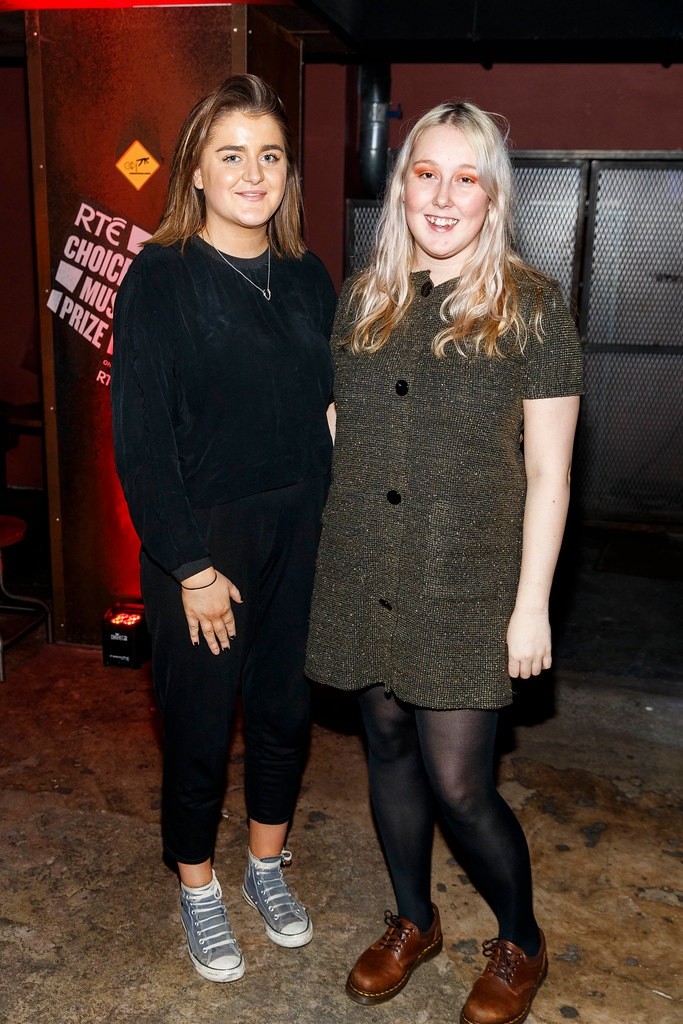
[299,91,586,1023]
[109,71,336,985]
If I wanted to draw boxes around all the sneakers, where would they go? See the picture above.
[242,850,312,948]
[177,871,244,984]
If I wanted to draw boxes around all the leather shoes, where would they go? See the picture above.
[460,927,549,1022]
[345,898,443,1004]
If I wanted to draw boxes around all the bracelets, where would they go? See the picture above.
[180,571,217,591]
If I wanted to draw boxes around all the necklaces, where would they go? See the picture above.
[204,219,273,302]
